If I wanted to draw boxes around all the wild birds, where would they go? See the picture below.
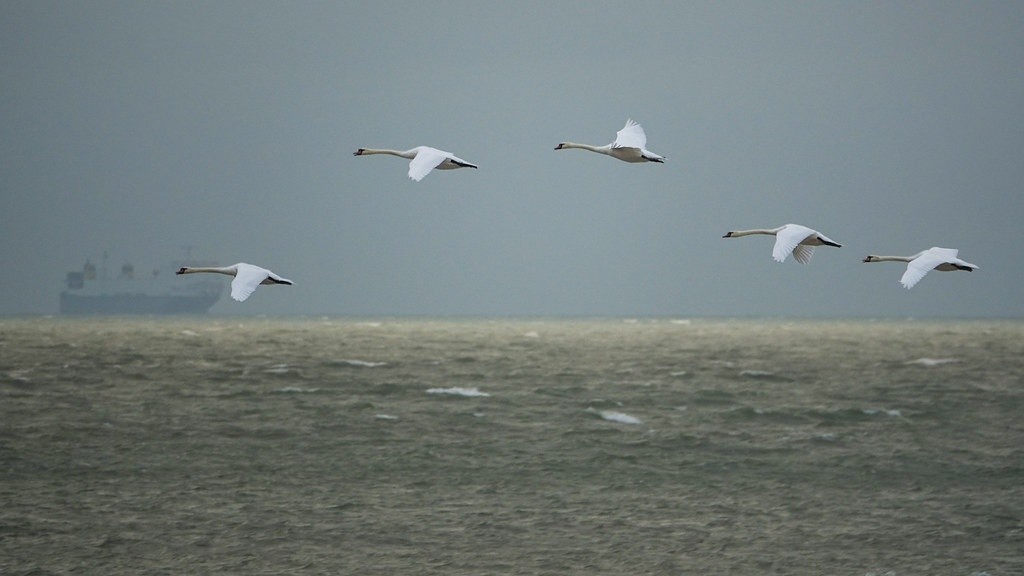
[721,223,846,265]
[863,246,979,291]
[174,262,294,304]
[352,144,478,183]
[554,118,668,164]
